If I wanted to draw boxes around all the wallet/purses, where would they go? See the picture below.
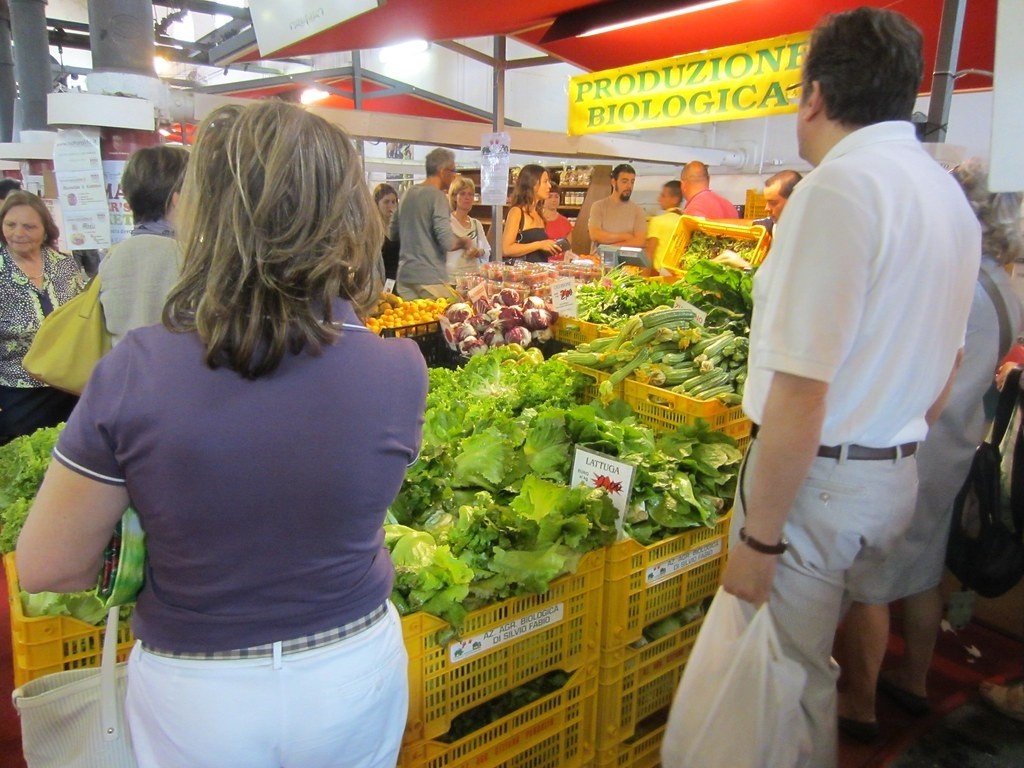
[543,238,571,257]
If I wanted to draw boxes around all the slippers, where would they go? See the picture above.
[980,680,1024,720]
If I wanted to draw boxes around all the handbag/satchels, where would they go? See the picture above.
[11,606,141,768]
[945,369,1024,595]
[660,586,812,768]
[22,242,120,396]
[502,204,526,265]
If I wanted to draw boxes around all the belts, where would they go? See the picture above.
[749,420,919,463]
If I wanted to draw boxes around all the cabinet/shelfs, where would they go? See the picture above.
[454,165,611,252]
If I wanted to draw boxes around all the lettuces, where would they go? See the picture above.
[382,345,742,746]
[0,421,135,668]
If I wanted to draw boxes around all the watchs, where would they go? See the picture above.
[739,529,787,556]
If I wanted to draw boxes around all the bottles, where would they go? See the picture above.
[560,191,587,206]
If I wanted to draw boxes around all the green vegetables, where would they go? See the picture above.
[576,231,758,328]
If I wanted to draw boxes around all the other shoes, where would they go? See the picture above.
[883,675,932,715]
[836,709,884,747]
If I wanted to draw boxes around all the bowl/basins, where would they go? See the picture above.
[447,260,603,310]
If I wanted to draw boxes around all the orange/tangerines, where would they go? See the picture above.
[366,292,448,338]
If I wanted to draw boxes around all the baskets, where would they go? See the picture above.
[4,550,138,688]
[379,215,773,768]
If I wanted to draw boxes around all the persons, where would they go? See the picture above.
[719,8,981,768]
[96,145,190,346]
[0,189,90,447]
[750,169,803,238]
[817,152,1021,734]
[371,146,737,302]
[15,101,427,767]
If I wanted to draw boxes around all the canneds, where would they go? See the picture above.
[564,191,587,207]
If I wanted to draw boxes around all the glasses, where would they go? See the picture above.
[458,192,474,199]
[449,167,458,173]
[785,71,824,100]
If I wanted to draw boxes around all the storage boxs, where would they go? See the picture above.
[370,213,771,458]
[401,501,735,768]
[3,548,138,692]
